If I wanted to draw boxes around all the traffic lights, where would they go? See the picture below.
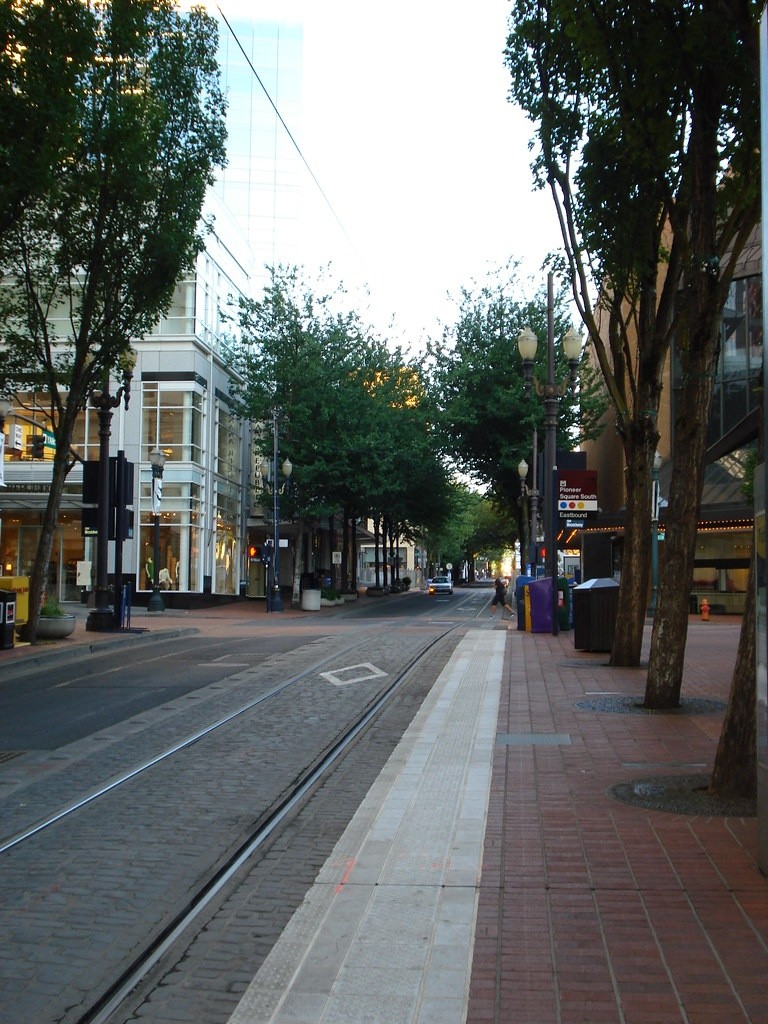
[31,434,45,458]
[248,545,261,562]
[152,463,164,477]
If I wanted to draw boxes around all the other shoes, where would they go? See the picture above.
[509,611,516,618]
[488,616,495,620]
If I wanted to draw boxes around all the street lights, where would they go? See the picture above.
[516,269,585,631]
[259,457,293,613]
[148,446,165,613]
[653,450,663,621]
[517,456,543,577]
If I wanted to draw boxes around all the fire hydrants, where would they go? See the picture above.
[700,599,711,622]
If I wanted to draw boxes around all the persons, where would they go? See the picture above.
[159,568,173,590]
[145,557,154,589]
[489,574,516,619]
[175,561,180,590]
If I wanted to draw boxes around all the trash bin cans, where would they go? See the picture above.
[302,577,323,611]
[573,578,620,652]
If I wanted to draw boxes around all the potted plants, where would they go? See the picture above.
[33,594,76,639]
[367,577,412,597]
[321,587,357,607]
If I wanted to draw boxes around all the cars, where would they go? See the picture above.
[428,576,455,594]
